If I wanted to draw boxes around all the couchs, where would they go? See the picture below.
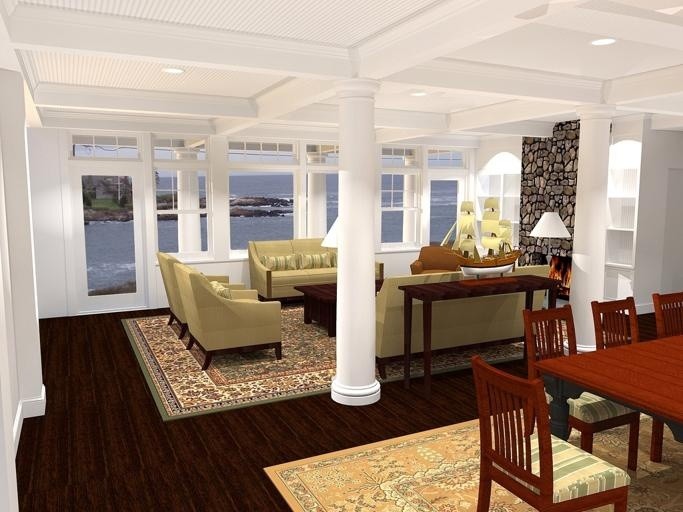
[374,265,550,380]
[248,238,384,300]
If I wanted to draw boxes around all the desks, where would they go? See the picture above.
[398,275,563,404]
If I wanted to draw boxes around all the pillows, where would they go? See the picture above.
[209,278,232,302]
[298,252,332,269]
[331,252,337,267]
[260,254,298,270]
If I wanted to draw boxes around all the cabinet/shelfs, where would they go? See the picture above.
[602,116,666,315]
[476,151,523,264]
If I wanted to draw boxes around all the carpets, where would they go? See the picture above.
[121,303,562,421]
[263,405,683,512]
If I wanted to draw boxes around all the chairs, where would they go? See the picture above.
[173,263,282,371]
[410,246,461,276]
[156,252,245,339]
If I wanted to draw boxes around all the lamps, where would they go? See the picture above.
[529,211,572,309]
[321,215,338,249]
